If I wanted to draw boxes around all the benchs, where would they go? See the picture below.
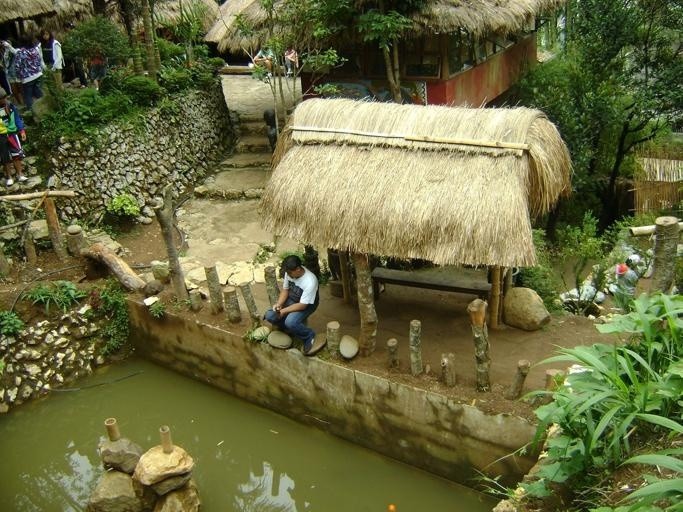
[370,266,492,304]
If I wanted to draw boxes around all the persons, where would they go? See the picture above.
[0,88,28,187]
[264,254,319,353]
[0,22,108,111]
[281,44,298,78]
[615,255,638,315]
[252,45,277,79]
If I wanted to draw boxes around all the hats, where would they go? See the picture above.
[0,86,7,99]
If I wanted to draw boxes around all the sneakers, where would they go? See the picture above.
[303,332,316,352]
[16,176,29,181]
[7,179,14,185]
